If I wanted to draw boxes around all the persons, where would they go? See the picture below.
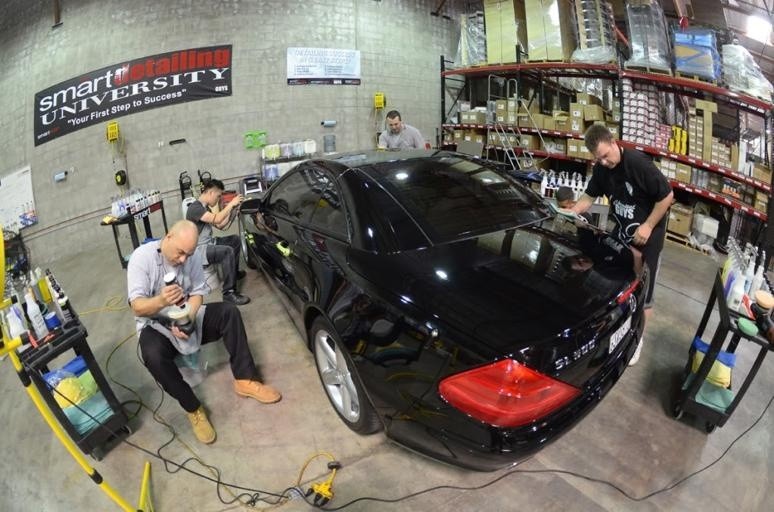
[550,184,598,226]
[125,220,282,446]
[556,123,673,366]
[185,178,250,305]
[379,110,426,153]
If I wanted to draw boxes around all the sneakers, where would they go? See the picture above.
[628,336,644,367]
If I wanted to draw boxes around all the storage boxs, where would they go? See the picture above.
[443,92,773,239]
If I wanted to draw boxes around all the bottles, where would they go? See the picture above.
[0,264,74,354]
[719,235,766,311]
[109,187,161,218]
[163,272,186,309]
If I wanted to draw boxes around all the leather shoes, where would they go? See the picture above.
[235,379,282,404]
[188,405,216,444]
[223,291,250,305]
[235,270,246,280]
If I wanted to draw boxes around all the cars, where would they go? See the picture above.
[232,146,646,474]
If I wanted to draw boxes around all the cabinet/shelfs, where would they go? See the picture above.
[99,200,169,269]
[670,265,774,433]
[8,297,133,462]
[438,54,774,273]
[259,153,314,188]
